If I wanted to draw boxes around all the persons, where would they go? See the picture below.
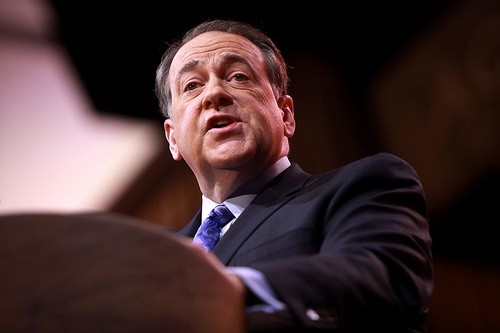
[1,19,435,333]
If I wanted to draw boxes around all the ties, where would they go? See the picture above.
[196,204,236,253]
